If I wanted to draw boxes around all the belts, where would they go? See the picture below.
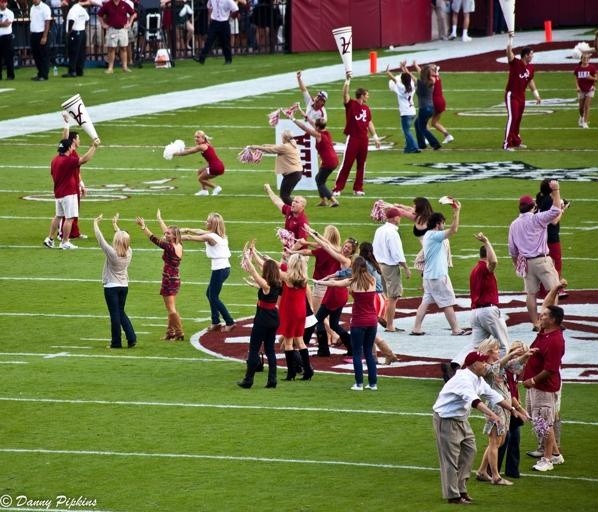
[477,304,491,308]
[526,254,550,259]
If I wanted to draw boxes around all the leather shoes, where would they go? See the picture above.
[447,472,513,504]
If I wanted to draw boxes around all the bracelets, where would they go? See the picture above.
[141,225,146,231]
[532,90,539,99]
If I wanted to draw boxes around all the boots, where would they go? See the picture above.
[170,313,184,340]
[160,314,176,340]
[236,349,313,388]
[313,332,397,365]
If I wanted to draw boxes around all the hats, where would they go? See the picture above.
[518,195,534,209]
[461,351,488,369]
[57,139,71,154]
[386,208,401,218]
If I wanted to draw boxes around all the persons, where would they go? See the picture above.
[55,110,88,241]
[573,55,598,129]
[246,60,454,199]
[43,139,100,250]
[135,208,185,341]
[503,33,541,152]
[431,0,450,42]
[172,130,225,196]
[0,0,292,83]
[235,178,569,507]
[450,0,476,43]
[92,213,136,350]
[179,212,235,334]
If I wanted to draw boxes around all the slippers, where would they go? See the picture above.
[450,330,472,335]
[384,327,404,332]
[408,332,425,335]
[378,318,387,328]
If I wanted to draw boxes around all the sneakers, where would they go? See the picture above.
[211,186,222,195]
[44,233,89,249]
[441,135,454,145]
[194,189,209,196]
[582,124,589,129]
[527,450,565,472]
[350,383,364,390]
[316,190,365,207]
[364,384,377,391]
[578,116,583,126]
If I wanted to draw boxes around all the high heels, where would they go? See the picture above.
[220,324,236,333]
[206,324,221,331]
